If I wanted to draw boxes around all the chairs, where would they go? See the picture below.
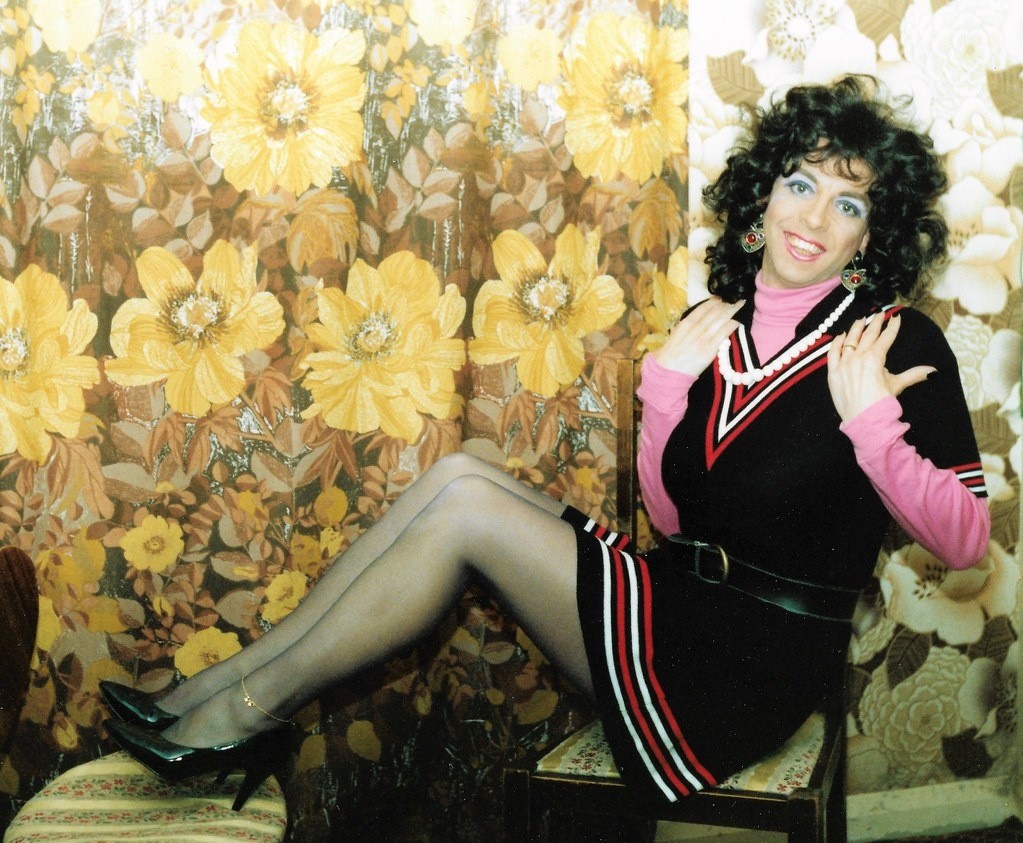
[0,544,294,843]
[486,335,876,843]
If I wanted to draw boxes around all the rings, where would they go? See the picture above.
[843,345,856,351]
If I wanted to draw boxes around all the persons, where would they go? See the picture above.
[98,72,991,808]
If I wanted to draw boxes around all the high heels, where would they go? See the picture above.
[102,718,295,812]
[98,680,236,794]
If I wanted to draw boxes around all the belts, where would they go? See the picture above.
[658,534,861,623]
